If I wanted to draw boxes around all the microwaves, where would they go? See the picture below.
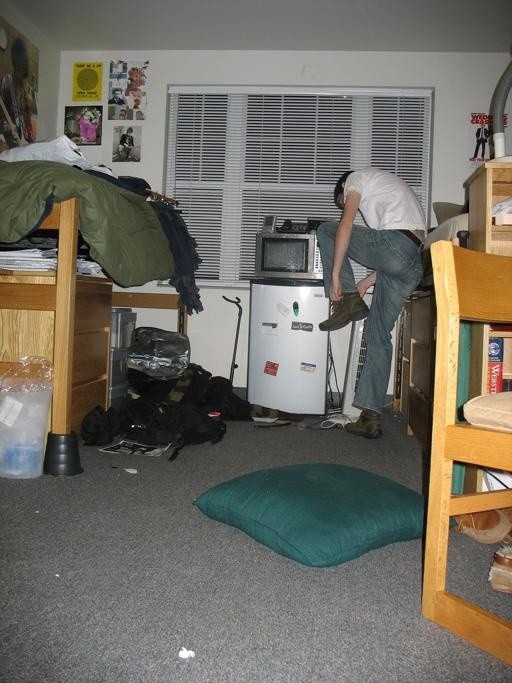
[254,232,325,279]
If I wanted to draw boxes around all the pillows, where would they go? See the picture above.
[192,462,459,569]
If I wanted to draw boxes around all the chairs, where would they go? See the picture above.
[421,239,512,666]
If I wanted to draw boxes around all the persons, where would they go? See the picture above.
[0,37,34,147]
[470,123,489,160]
[120,127,135,159]
[108,61,146,120]
[316,168,428,440]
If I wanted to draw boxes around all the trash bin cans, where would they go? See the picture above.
[0,377,53,479]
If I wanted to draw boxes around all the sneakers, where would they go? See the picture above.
[319,292,370,331]
[345,410,383,439]
[453,507,512,595]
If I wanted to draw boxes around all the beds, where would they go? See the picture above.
[1,175,190,459]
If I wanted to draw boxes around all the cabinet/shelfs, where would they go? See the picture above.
[1,270,114,455]
[462,162,512,496]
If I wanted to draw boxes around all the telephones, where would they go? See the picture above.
[278,219,309,234]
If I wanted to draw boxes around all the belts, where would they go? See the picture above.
[400,229,424,249]
